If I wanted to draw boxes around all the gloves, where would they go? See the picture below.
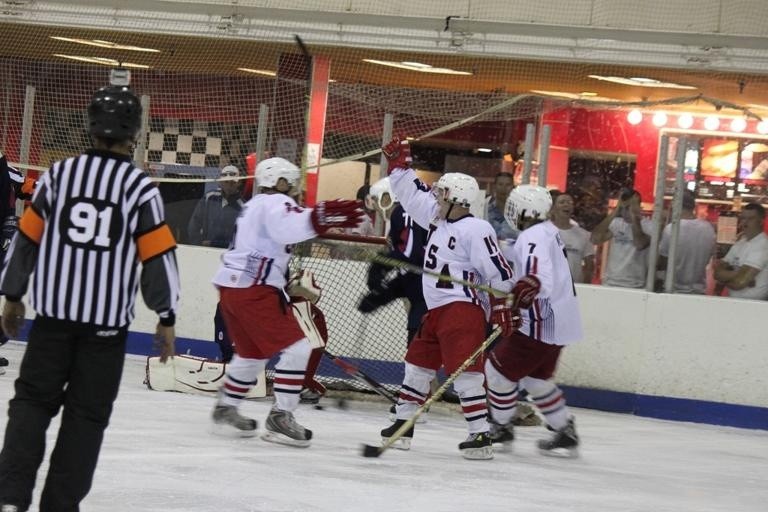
[382,137,411,176]
[509,276,540,312]
[312,198,366,236]
[490,292,523,334]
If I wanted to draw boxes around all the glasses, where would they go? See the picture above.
[220,172,236,177]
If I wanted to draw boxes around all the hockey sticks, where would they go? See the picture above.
[361,222,509,302]
[357,326,501,456]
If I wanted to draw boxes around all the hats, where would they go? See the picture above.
[217,166,239,184]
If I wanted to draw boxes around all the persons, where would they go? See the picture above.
[0,148,39,367]
[356,174,452,414]
[209,143,364,444]
[486,171,768,304]
[481,183,582,453]
[379,134,519,448]
[0,87,183,509]
[191,165,247,249]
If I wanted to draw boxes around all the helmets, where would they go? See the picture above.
[254,157,300,187]
[504,185,554,231]
[88,88,143,141]
[430,172,479,208]
[370,177,399,211]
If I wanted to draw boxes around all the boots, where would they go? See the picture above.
[213,405,256,430]
[300,384,322,400]
[381,398,576,449]
[267,408,312,441]
